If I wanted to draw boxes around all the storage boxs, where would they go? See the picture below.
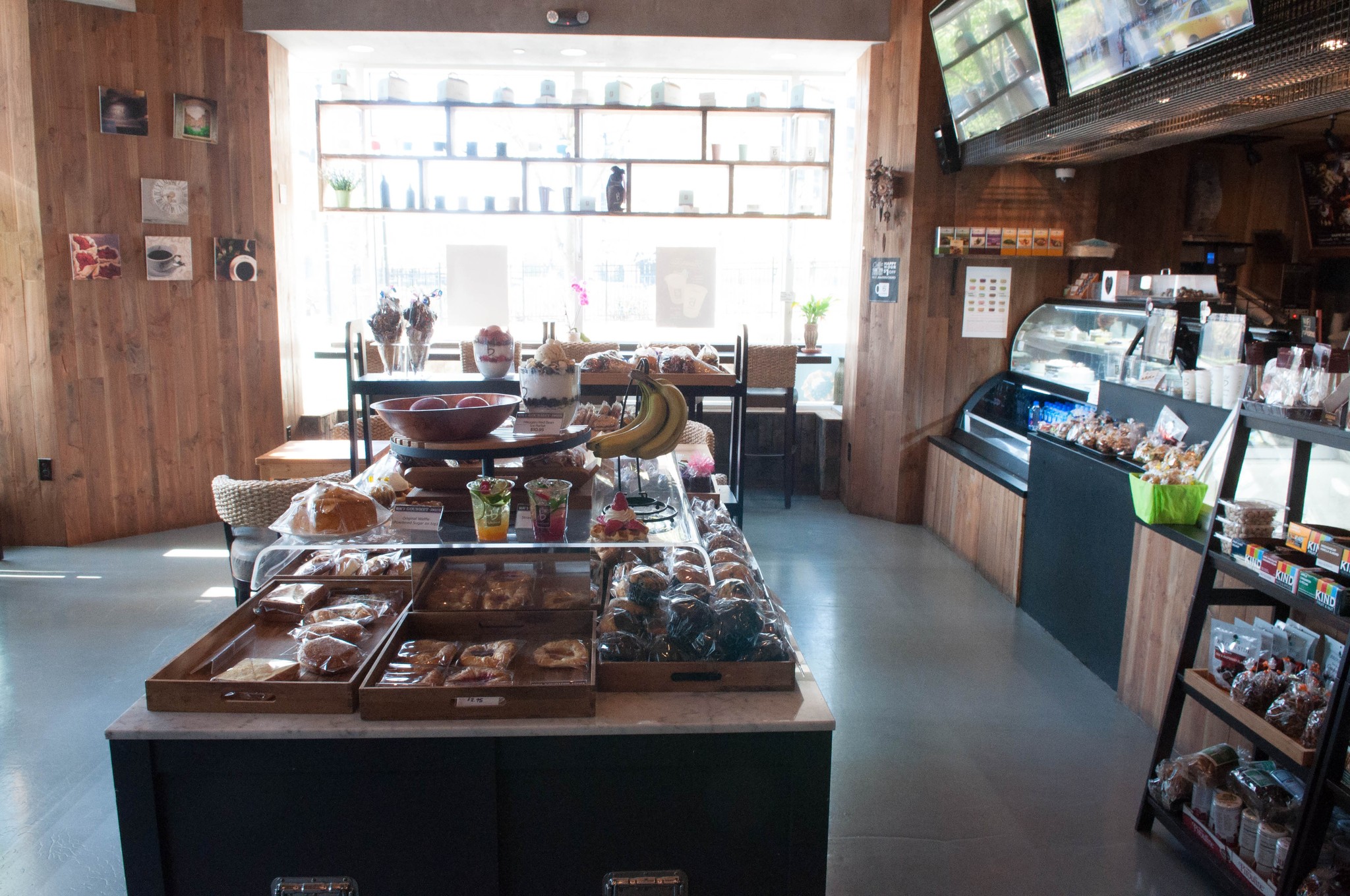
[1101,270,1129,302]
[933,226,1065,255]
[1129,473,1350,616]
[144,542,796,720]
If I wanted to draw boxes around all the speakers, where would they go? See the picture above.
[934,124,961,174]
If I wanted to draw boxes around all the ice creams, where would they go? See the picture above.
[368,286,437,378]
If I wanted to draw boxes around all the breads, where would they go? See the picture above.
[570,401,636,437]
[380,570,589,686]
[593,508,789,660]
[1037,416,1207,486]
[582,344,725,376]
[288,456,458,535]
[617,461,712,493]
[522,446,587,469]
[209,546,413,680]
[1152,671,1350,896]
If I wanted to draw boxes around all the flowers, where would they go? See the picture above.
[564,279,591,343]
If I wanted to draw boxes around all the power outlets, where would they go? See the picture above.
[38,458,52,480]
[287,426,291,440]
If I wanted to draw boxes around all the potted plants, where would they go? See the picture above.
[794,297,829,353]
[328,174,353,208]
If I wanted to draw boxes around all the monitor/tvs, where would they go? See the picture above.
[928,0,1051,146]
[1053,0,1254,97]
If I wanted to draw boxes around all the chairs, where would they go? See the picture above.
[211,470,352,606]
[334,415,394,440]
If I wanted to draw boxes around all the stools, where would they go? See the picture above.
[652,343,703,423]
[459,340,520,375]
[559,343,624,406]
[744,345,799,509]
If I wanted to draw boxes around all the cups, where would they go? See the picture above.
[485,196,495,211]
[805,147,816,162]
[712,143,720,161]
[739,144,748,161]
[466,142,478,158]
[435,195,445,209]
[234,261,255,281]
[466,479,515,541]
[496,142,506,157]
[518,364,581,430]
[1181,362,1249,409]
[770,146,780,161]
[508,196,520,211]
[473,335,515,378]
[146,244,182,272]
[524,479,573,543]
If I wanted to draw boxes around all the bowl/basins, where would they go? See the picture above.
[1129,472,1208,524]
[369,393,525,441]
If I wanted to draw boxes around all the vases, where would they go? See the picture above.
[566,332,580,343]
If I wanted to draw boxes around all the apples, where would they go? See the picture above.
[409,396,490,410]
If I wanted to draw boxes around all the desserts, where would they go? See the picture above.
[519,322,580,430]
[70,234,121,281]
[473,325,513,379]
[590,492,649,541]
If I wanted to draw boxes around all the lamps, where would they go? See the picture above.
[546,9,590,26]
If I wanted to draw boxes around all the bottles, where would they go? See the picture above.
[381,175,391,209]
[406,186,415,209]
[563,187,572,212]
[538,186,550,211]
[1028,400,1096,432]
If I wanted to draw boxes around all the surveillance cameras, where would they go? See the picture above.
[1056,167,1076,183]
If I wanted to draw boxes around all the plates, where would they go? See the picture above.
[229,254,258,281]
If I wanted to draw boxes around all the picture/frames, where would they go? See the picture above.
[70,85,256,282]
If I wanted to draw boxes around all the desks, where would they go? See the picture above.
[315,349,832,364]
[255,440,390,481]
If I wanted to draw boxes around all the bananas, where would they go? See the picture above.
[585,369,688,460]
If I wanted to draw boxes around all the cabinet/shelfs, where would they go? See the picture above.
[110,731,832,896]
[951,298,1232,485]
[315,100,835,219]
[344,320,750,530]
[1135,368,1350,896]
